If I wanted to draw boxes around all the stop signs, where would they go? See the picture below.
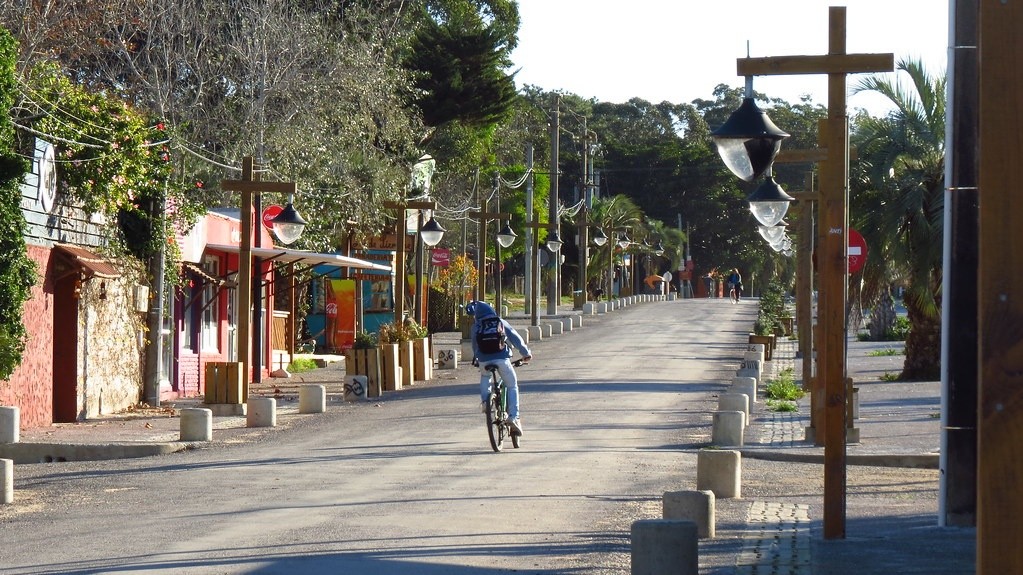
[849,226,868,273]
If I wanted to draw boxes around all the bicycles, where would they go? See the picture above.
[474,358,528,457]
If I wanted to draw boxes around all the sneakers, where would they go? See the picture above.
[481,401,493,414]
[506,416,523,437]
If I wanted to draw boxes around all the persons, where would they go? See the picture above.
[589,276,602,303]
[730,268,742,301]
[472,301,532,436]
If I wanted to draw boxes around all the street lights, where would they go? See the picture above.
[466,198,518,302]
[523,205,560,327]
[380,185,446,346]
[607,218,636,301]
[714,0,897,540]
[571,210,605,309]
[642,236,664,279]
[213,153,305,402]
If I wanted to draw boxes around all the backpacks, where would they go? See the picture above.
[474,317,506,354]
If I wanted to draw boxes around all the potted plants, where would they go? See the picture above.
[748,276,793,361]
[346,321,432,396]
